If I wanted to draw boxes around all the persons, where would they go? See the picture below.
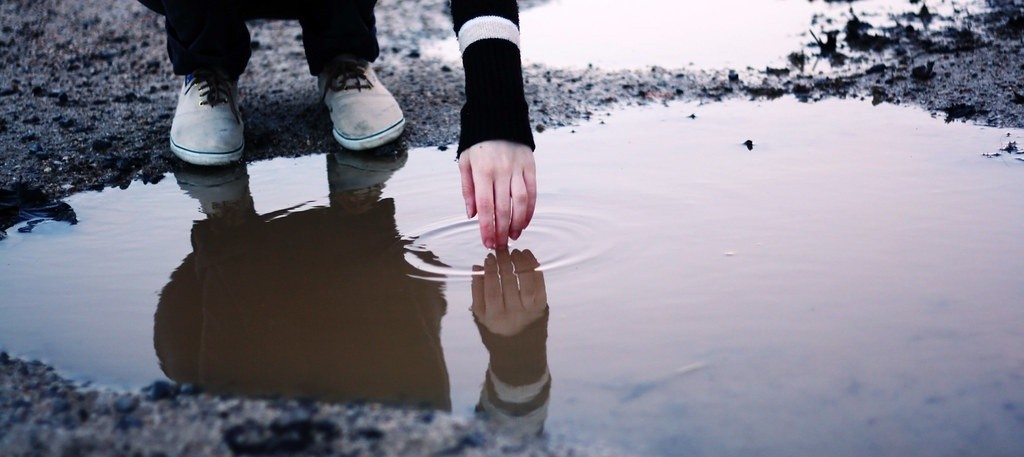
[156,152,552,438]
[137,0,536,248]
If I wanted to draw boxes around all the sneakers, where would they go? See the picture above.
[328,148,407,191]
[176,166,250,213]
[317,54,406,151]
[169,63,245,166]
